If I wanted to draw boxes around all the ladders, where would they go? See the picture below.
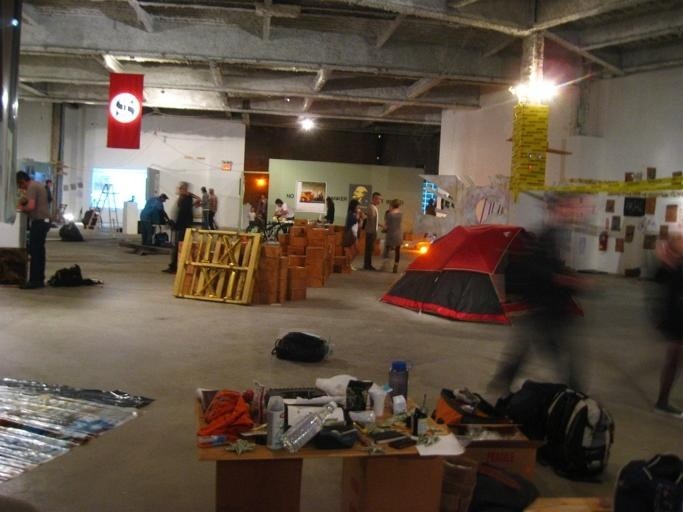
[86,183,120,235]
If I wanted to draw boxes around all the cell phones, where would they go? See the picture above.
[389,437,417,449]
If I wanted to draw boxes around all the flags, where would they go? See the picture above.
[107,72,144,149]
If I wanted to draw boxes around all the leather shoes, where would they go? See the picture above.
[20,282,42,288]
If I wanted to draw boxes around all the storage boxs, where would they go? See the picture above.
[176,219,382,309]
[446,420,547,493]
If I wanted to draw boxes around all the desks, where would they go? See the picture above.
[522,496,616,512]
[196,394,464,512]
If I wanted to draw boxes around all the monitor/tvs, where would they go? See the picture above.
[624,197,646,216]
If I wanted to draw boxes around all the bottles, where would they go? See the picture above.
[279,400,338,453]
[265,395,284,450]
[388,361,408,402]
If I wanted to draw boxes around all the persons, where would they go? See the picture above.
[486,184,595,401]
[648,226,682,418]
[16,171,51,288]
[135,182,403,274]
[44,180,54,222]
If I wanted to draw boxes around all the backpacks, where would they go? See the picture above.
[272,332,327,362]
[545,388,613,478]
[614,454,683,512]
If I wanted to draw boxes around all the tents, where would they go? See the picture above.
[376,223,586,328]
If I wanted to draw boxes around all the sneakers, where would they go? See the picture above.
[654,404,682,419]
